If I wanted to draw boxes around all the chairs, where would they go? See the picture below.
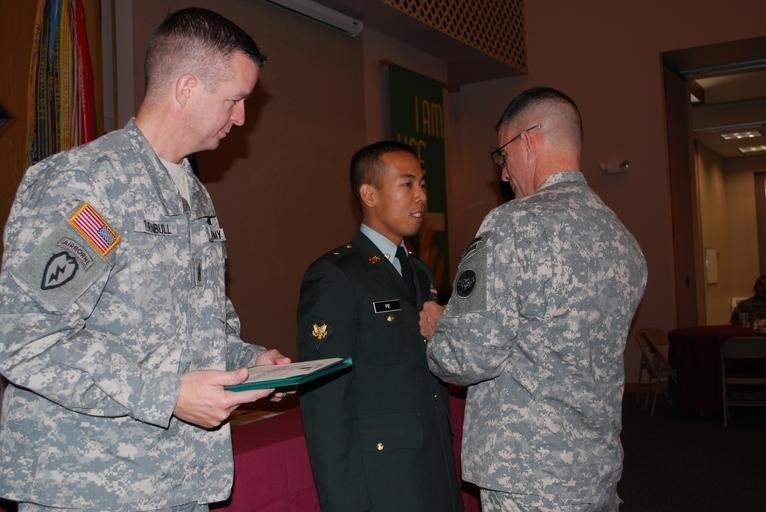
[626,326,766,431]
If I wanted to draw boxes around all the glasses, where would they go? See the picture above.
[490,123,539,167]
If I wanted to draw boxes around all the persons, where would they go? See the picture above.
[296,137,472,510]
[730,275,765,326]
[417,84,650,510]
[0,0,301,510]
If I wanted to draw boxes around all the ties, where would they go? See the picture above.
[395,246,414,291]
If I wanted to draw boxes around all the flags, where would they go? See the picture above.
[20,0,100,174]
